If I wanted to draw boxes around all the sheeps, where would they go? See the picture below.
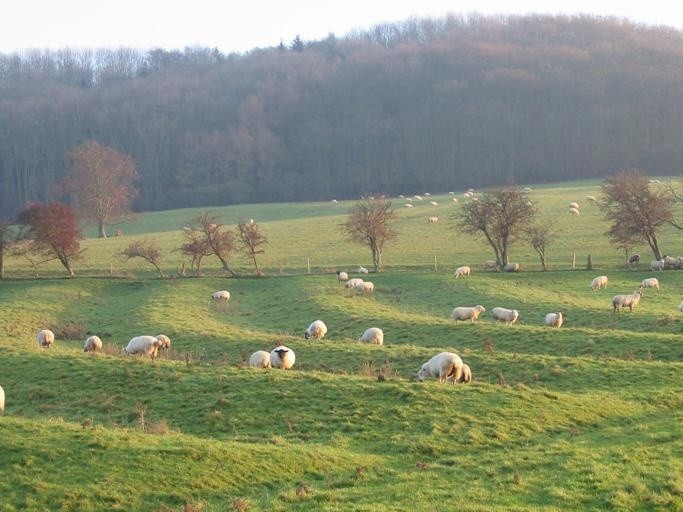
[545,312,565,328]
[484,260,500,272]
[359,327,383,345]
[210,291,230,300]
[612,290,644,312]
[331,187,596,224]
[123,336,163,360]
[250,351,272,368]
[417,352,463,385]
[654,180,659,183]
[155,334,170,360]
[492,307,519,326]
[336,265,374,295]
[649,255,683,272]
[304,320,327,340]
[450,305,486,325]
[443,363,471,384]
[454,267,470,279]
[269,345,295,370]
[639,277,659,292]
[503,263,519,272]
[0,385,5,417]
[83,336,102,354]
[588,275,608,292]
[630,254,640,264]
[648,180,654,183]
[36,330,54,348]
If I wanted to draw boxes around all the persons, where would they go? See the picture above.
[585,253,592,270]
